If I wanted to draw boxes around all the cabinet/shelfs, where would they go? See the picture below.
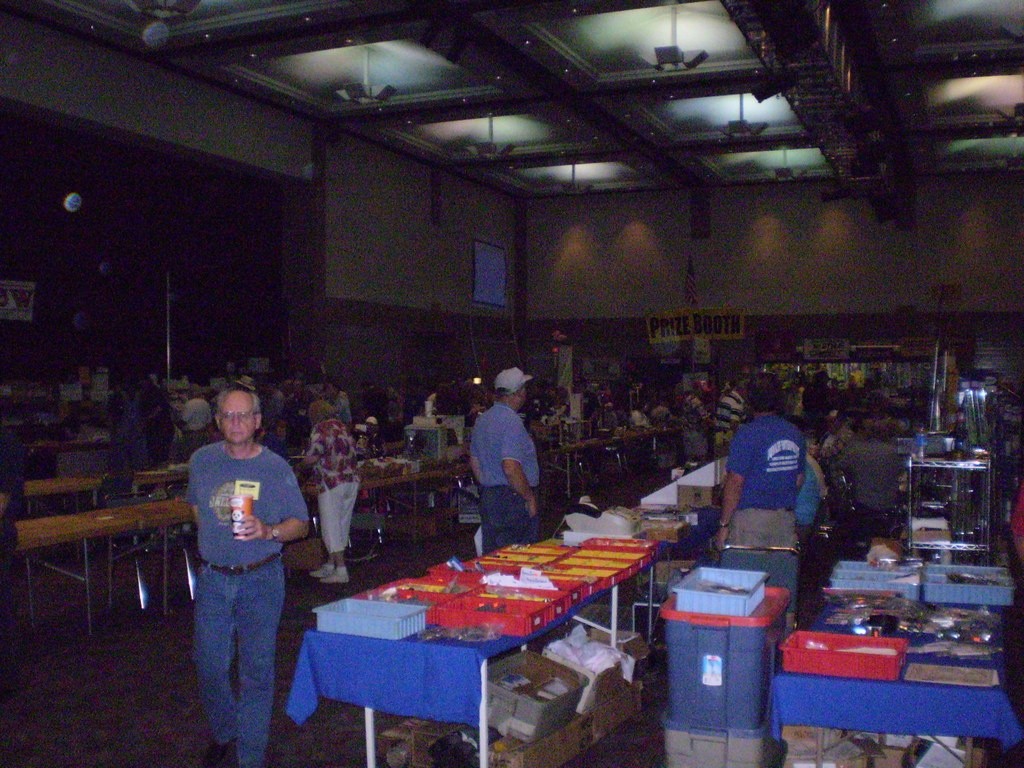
[908,454,990,565]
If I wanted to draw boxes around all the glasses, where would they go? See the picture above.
[218,411,253,420]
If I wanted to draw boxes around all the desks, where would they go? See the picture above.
[772,605,1024,768]
[0,414,680,639]
[285,503,720,768]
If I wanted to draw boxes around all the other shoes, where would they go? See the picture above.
[309,567,335,578]
[319,572,349,583]
[203,743,234,766]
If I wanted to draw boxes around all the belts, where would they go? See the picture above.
[200,553,276,575]
[754,506,794,512]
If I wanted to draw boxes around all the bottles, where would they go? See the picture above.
[915,422,927,459]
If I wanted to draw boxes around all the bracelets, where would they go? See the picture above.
[718,520,730,528]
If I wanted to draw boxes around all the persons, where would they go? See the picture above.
[0,367,256,705]
[799,371,1024,593]
[469,367,541,551]
[295,400,363,584]
[708,370,806,631]
[260,359,495,464]
[183,387,310,768]
[532,363,755,462]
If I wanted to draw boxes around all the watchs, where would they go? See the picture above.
[270,524,279,541]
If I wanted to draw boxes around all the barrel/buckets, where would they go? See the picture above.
[662,585,791,729]
[660,705,769,768]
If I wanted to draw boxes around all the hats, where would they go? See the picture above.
[494,367,534,395]
[235,375,256,391]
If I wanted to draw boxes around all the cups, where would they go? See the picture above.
[227,495,254,539]
[424,400,434,416]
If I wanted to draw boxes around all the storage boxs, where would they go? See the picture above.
[312,478,1017,768]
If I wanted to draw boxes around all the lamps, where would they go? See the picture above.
[128,0,1024,168]
